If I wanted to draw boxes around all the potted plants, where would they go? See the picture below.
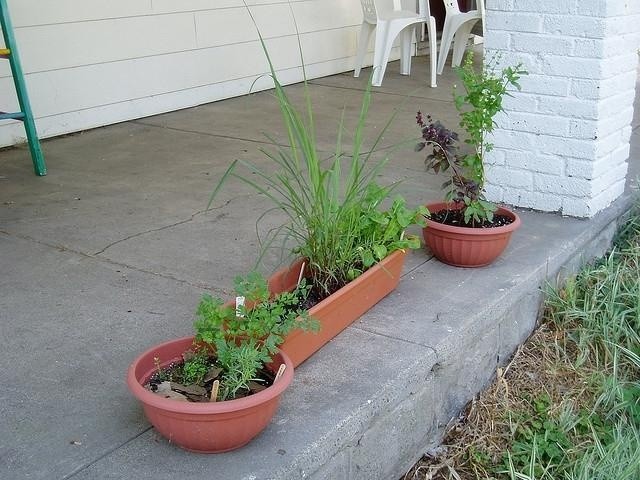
[128,271,319,452]
[206,4,430,371]
[414,38,528,267]
